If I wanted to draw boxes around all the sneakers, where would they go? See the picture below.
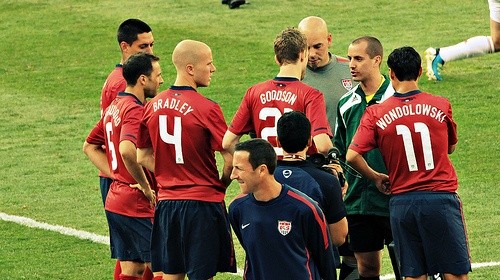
[425,47,445,81]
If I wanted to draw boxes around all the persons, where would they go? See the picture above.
[332,36,402,280]
[98,18,154,280]
[136,39,237,280]
[294,15,360,135]
[83,52,164,280]
[345,46,474,280]
[423,0,499,83]
[228,138,338,280]
[222,25,349,200]
[274,111,348,280]
